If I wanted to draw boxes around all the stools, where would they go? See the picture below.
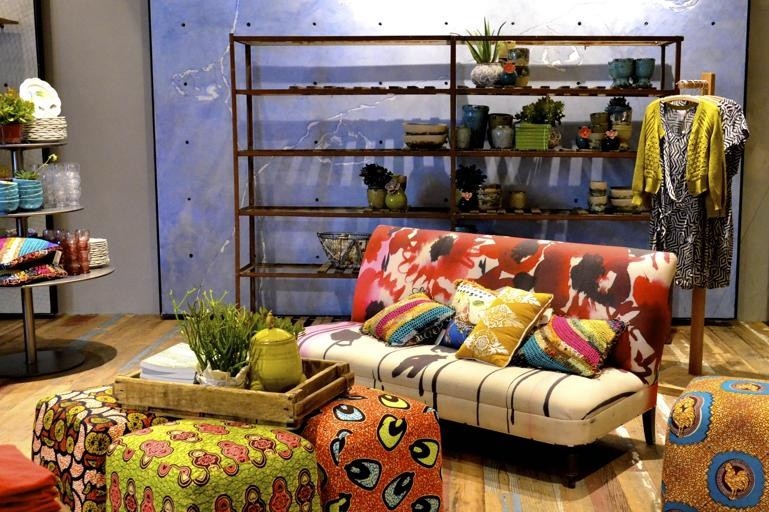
[107,418,322,512]
[31,386,192,511]
[660,372,768,510]
[298,383,446,510]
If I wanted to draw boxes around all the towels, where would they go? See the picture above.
[1,445,60,510]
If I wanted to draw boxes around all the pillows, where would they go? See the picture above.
[513,314,622,379]
[457,285,553,367]
[362,292,454,347]
[441,278,494,347]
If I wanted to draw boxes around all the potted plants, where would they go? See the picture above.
[360,164,389,210]
[516,96,565,147]
[169,287,306,389]
[383,174,408,210]
[606,95,634,139]
[1,87,35,142]
[450,163,486,211]
[462,19,506,87]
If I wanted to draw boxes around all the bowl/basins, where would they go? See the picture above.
[0,176,45,214]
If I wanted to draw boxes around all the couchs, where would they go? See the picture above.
[294,222,677,490]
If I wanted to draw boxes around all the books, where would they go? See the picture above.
[139,341,216,384]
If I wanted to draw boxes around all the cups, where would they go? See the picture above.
[43,225,91,278]
[42,161,83,211]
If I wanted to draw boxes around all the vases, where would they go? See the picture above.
[634,58,658,89]
[476,182,500,211]
[319,232,371,269]
[586,180,609,212]
[448,125,469,149]
[503,184,529,213]
[507,47,530,65]
[508,65,530,89]
[607,56,636,89]
[403,120,447,153]
[514,122,552,151]
[486,113,515,151]
[578,113,619,153]
[609,186,636,211]
[460,104,488,151]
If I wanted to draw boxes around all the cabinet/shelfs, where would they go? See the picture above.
[0,142,115,379]
[656,78,723,106]
[227,32,685,327]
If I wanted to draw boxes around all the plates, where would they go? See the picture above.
[87,236,111,270]
[19,74,62,117]
[22,114,68,142]
